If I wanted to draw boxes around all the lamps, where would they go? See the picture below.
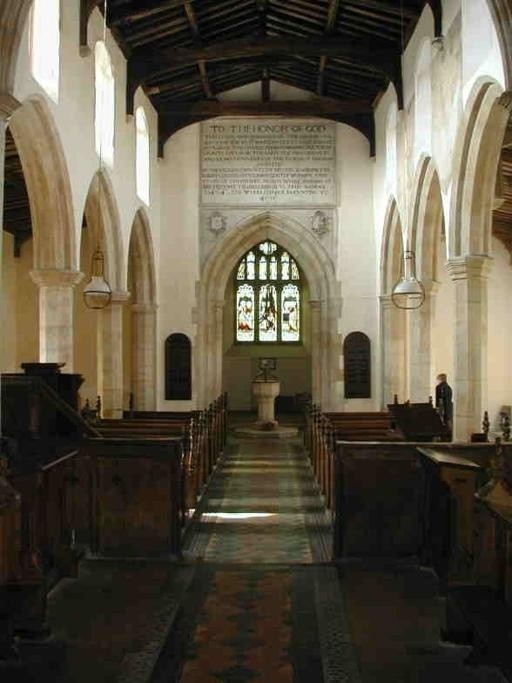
[83,1,111,309]
[392,0,426,310]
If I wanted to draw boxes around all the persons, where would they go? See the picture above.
[435,373,453,421]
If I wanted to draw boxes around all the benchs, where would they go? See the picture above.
[303,394,473,555]
[80,393,238,560]
